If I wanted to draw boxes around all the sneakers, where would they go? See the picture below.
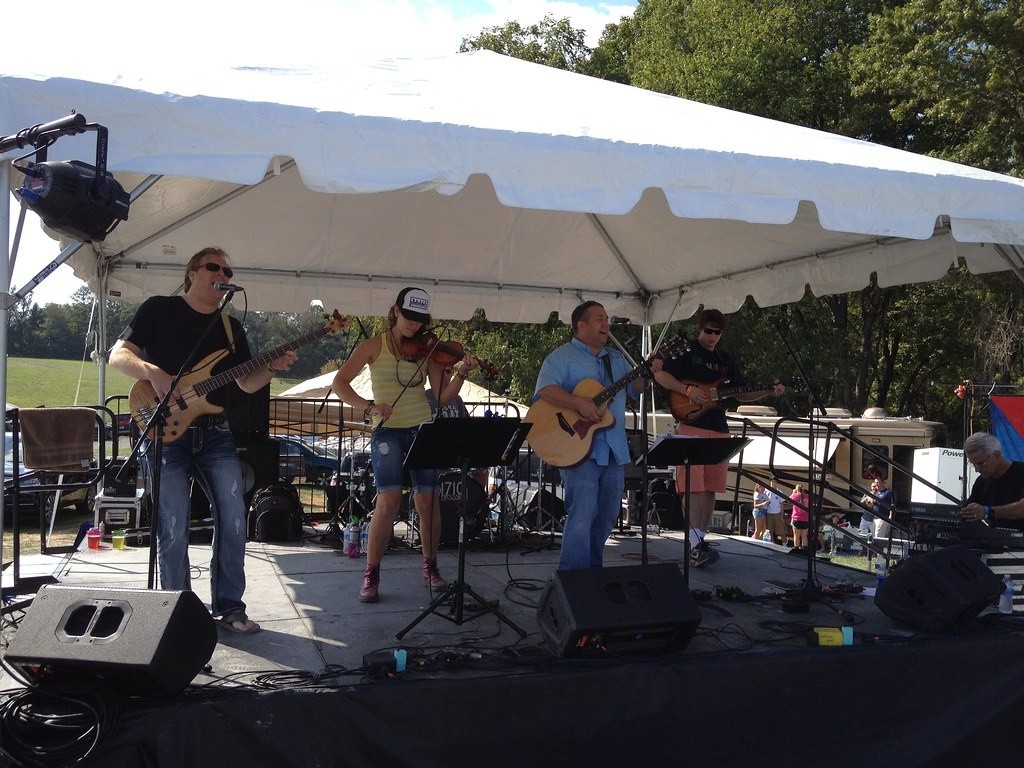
[689,539,721,567]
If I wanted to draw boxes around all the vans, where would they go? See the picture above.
[486,449,566,523]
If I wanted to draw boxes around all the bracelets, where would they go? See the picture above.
[456,372,468,380]
[984,506,989,518]
[366,403,375,414]
[685,385,691,397]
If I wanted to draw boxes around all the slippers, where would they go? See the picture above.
[213,609,261,634]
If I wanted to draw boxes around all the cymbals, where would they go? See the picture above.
[332,421,372,433]
[484,395,532,399]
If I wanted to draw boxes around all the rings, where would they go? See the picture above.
[467,363,471,367]
[974,514,977,519]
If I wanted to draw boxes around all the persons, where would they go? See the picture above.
[752,478,787,548]
[832,514,846,524]
[108,247,299,633]
[332,286,478,603]
[533,301,662,570]
[857,477,894,558]
[788,485,826,553]
[654,308,785,567]
[960,433,1024,521]
[426,365,497,528]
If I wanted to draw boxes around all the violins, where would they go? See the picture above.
[400,328,500,376]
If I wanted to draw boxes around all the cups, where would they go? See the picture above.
[111,529,127,551]
[87,531,101,550]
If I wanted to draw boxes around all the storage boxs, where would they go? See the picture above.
[93,489,148,539]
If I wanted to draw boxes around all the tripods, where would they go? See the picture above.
[469,445,562,555]
[394,416,526,641]
[326,436,371,533]
[746,325,854,621]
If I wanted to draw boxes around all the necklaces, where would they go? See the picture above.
[391,331,422,361]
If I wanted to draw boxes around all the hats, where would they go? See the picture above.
[396,287,431,325]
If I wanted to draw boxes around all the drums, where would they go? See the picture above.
[409,470,490,546]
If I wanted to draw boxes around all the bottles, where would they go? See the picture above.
[763,530,771,543]
[99,521,106,542]
[998,574,1013,614]
[875,553,888,585]
[343,515,371,558]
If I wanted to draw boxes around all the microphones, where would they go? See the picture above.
[764,313,784,326]
[465,520,474,525]
[610,316,631,325]
[355,316,369,340]
[214,280,244,291]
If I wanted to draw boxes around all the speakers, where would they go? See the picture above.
[3,583,219,700]
[874,543,1006,635]
[536,562,702,660]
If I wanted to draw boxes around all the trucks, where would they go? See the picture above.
[623,406,952,535]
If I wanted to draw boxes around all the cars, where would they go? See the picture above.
[2,403,97,527]
[94,423,113,440]
[270,433,374,489]
[114,413,131,436]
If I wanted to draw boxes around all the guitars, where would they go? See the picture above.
[524,338,692,470]
[128,308,355,445]
[667,376,811,424]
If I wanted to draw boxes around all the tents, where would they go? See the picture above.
[0,50,1024,521]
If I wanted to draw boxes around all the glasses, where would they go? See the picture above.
[445,369,453,374]
[701,328,722,335]
[192,262,234,279]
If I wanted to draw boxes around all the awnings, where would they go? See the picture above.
[729,434,840,470]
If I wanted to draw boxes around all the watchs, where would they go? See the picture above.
[267,362,278,374]
[989,506,995,520]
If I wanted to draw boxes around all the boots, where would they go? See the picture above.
[423,557,446,592]
[359,564,380,602]
